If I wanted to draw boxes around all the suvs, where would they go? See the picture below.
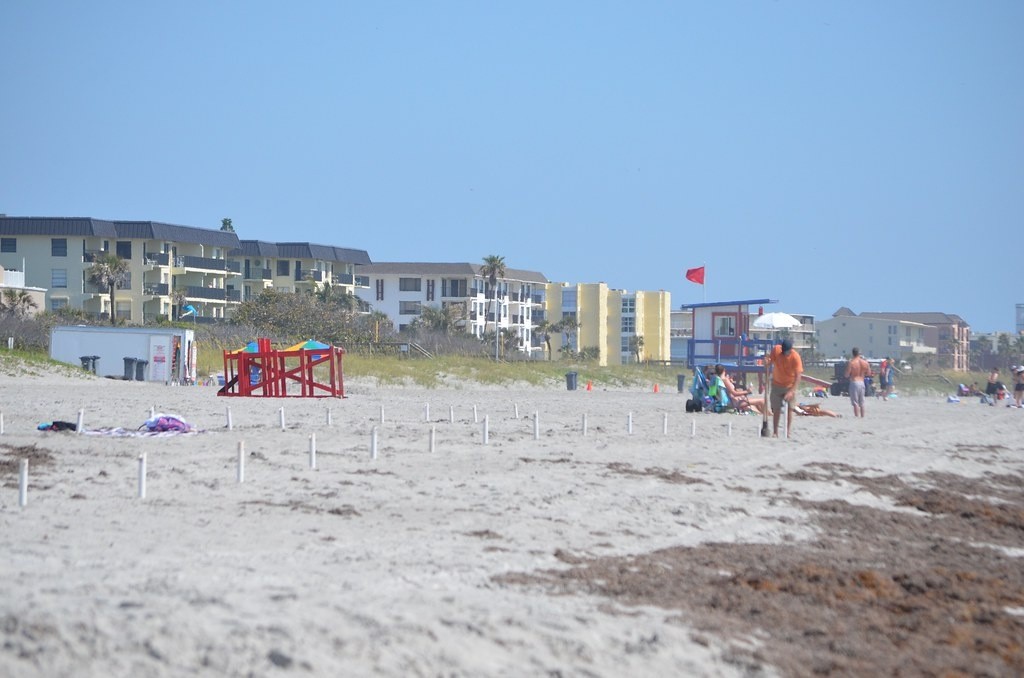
[829,362,876,397]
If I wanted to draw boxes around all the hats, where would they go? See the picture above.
[1017,366,1024,373]
[1012,366,1016,369]
[782,340,792,355]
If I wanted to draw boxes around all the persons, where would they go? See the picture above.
[962,382,978,395]
[987,368,1008,404]
[864,357,901,401]
[764,339,803,437]
[795,402,841,417]
[1011,365,1024,408]
[844,348,871,417]
[703,364,772,416]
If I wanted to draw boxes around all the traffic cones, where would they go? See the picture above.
[654,384,660,391]
[586,380,593,390]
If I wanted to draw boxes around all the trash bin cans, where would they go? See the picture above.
[677,375,686,393]
[123,356,137,380]
[79,355,101,375]
[565,371,577,390]
[137,359,149,381]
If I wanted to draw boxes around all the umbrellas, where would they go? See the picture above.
[754,312,802,340]
[285,338,330,359]
[226,341,258,354]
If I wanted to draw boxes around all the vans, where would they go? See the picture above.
[893,360,912,371]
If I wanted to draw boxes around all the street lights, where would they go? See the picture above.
[495,297,504,361]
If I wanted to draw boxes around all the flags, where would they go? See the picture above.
[686,266,704,285]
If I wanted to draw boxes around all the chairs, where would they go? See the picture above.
[689,366,719,413]
[709,374,754,416]
